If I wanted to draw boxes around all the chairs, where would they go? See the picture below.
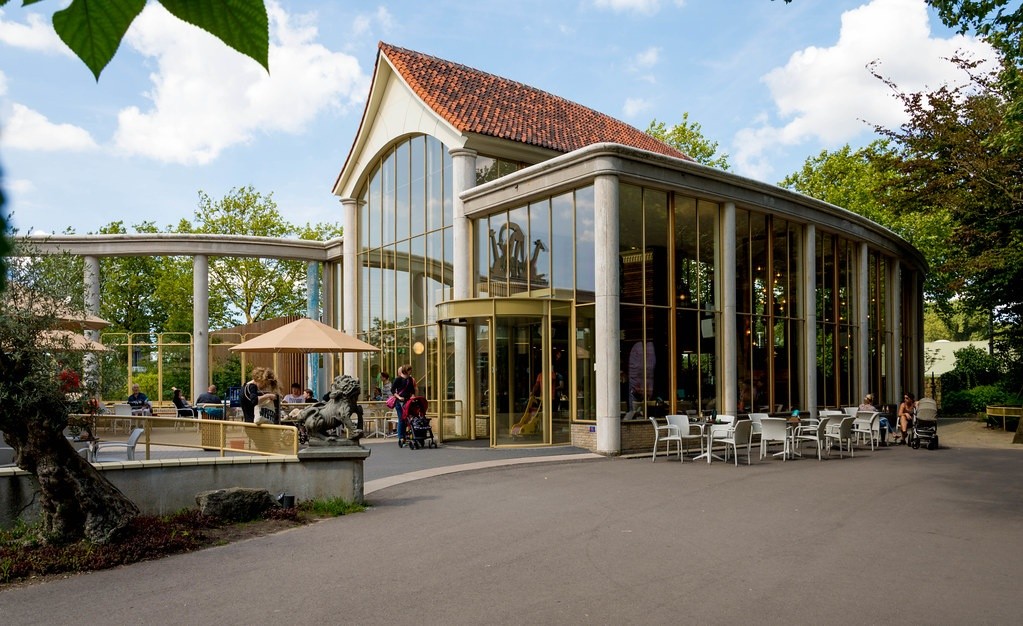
[114,404,131,434]
[649,405,900,467]
[94,428,144,462]
[172,402,195,433]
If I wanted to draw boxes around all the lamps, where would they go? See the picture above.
[276,492,294,511]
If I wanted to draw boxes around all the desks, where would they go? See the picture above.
[773,421,807,457]
[689,421,731,462]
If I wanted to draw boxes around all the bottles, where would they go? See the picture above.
[886,406,889,413]
[882,406,885,412]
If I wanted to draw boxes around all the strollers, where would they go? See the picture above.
[511,393,543,441]
[397,396,437,450]
[907,397,939,450]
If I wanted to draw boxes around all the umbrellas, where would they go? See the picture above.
[226,315,383,352]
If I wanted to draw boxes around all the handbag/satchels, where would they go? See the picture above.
[386,395,396,409]
[254,394,276,425]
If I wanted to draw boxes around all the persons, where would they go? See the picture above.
[859,394,902,447]
[366,364,420,449]
[172,367,319,425]
[897,392,917,445]
[126,385,155,414]
[57,359,80,392]
[739,380,757,413]
[530,364,563,431]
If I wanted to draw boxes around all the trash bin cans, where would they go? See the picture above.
[201,408,225,451]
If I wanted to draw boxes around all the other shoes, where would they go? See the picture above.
[903,431,908,438]
[901,440,906,445]
[893,435,901,440]
